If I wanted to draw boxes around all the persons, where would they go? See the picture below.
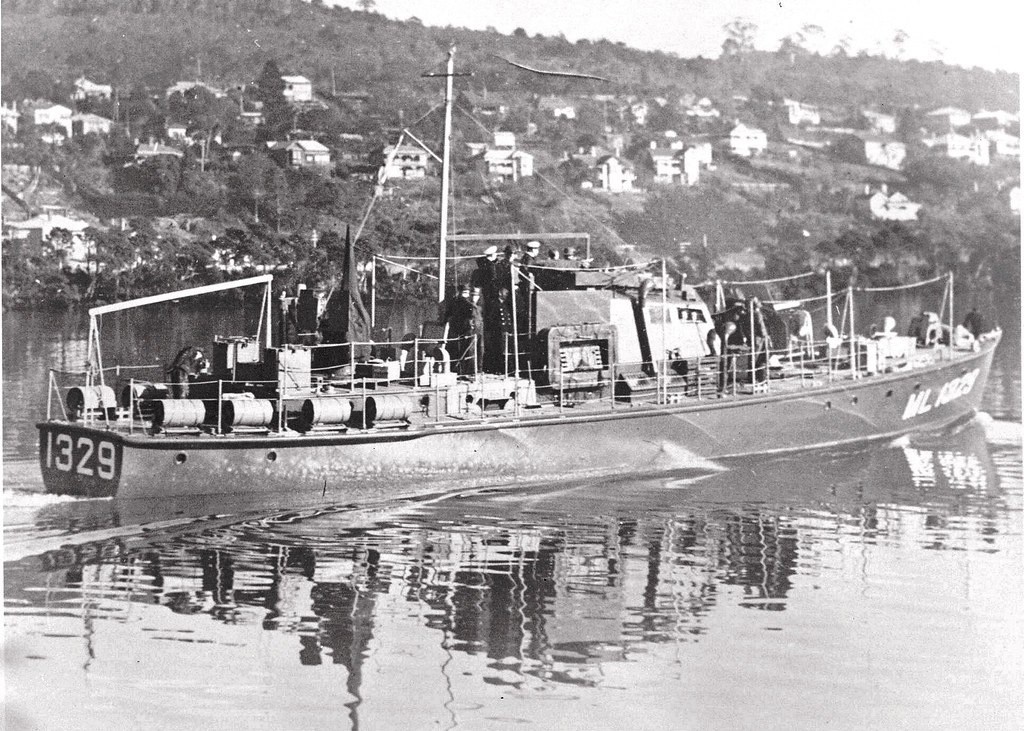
[445,241,576,374]
[963,307,985,339]
[741,296,768,385]
[166,347,203,400]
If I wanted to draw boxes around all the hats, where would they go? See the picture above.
[503,245,518,254]
[457,285,471,291]
[483,246,498,257]
[527,241,541,249]
[470,286,483,295]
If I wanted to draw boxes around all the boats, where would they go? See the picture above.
[34,41,1004,499]
